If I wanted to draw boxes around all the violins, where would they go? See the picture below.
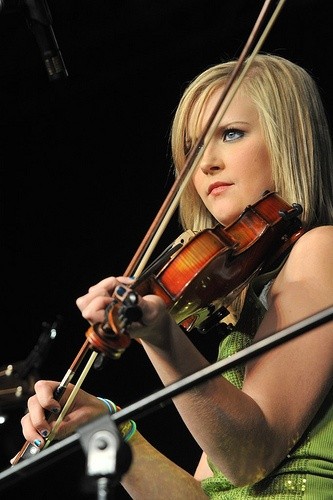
[86,190,306,356]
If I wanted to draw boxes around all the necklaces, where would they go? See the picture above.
[96,396,136,444]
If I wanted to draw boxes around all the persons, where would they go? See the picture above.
[21,56,333,500]
